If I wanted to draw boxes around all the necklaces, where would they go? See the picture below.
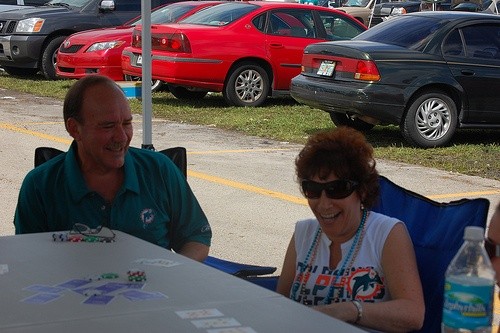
[291,208,368,306]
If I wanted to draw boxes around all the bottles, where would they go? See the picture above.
[441,227,496,333]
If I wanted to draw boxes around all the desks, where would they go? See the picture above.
[0,229,370,333]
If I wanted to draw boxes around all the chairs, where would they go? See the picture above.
[244,176,491,333]
[34,146,277,275]
[290,26,307,36]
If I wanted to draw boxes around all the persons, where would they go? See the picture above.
[278,126,424,333]
[12,75,214,262]
[485,201,500,333]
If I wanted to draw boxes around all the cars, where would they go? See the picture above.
[122,1,369,108]
[0,0,53,12]
[296,0,500,32]
[0,0,170,82]
[289,11,500,148]
[55,0,227,95]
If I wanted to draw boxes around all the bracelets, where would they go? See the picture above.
[351,301,362,324]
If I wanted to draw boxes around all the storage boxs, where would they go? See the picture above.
[117,81,141,100]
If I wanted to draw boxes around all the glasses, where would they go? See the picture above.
[69,223,115,240]
[301,179,361,200]
